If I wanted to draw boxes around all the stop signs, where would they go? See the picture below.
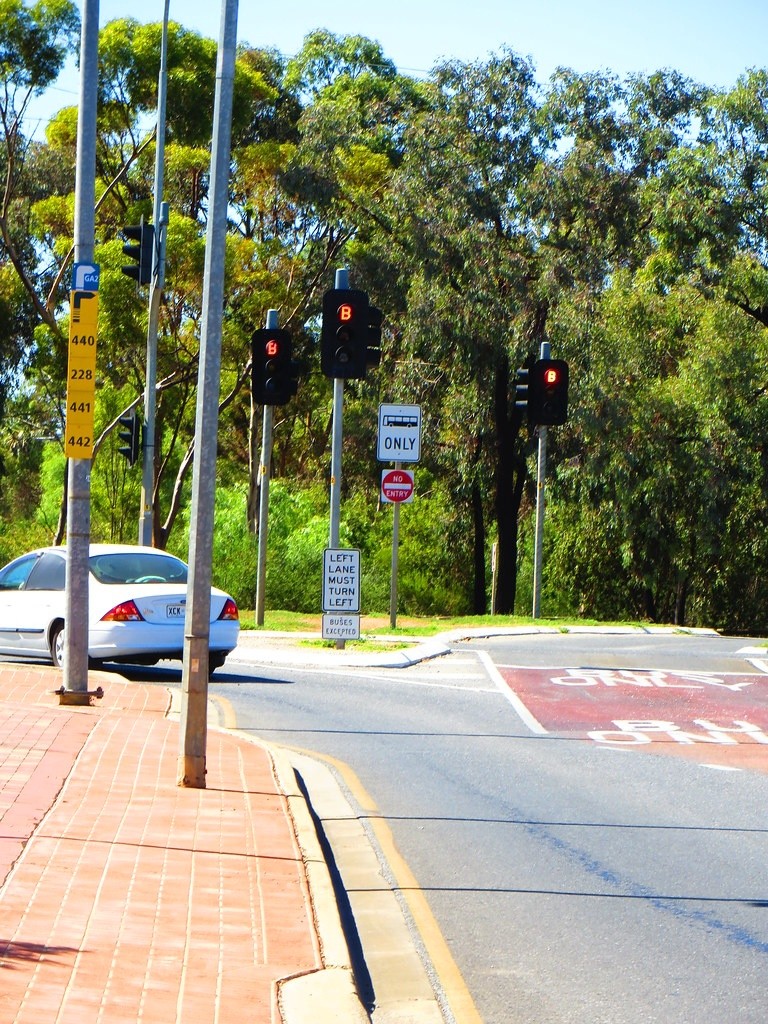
[382,470,414,503]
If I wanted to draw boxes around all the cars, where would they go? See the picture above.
[0,543,241,676]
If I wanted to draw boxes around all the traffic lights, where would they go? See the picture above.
[530,359,569,426]
[119,212,158,285]
[119,413,141,465]
[514,368,532,413]
[251,330,292,405]
[321,288,368,380]
[366,306,384,368]
